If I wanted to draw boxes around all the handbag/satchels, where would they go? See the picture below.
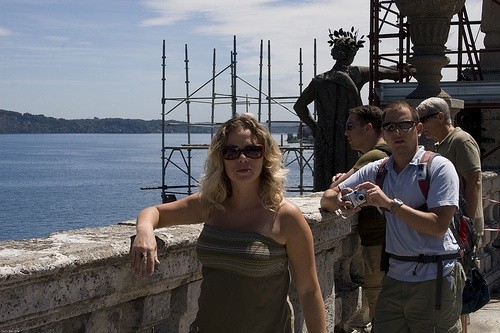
[461,263,491,314]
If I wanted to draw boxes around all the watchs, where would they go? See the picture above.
[390,198,404,216]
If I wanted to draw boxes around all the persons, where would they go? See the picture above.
[416,97,484,333]
[320,102,467,333]
[329,105,391,333]
[130,114,326,333]
[293,27,410,193]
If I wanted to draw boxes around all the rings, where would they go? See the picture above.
[140,253,147,258]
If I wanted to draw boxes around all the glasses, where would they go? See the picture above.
[381,120,418,131]
[419,111,442,123]
[221,143,264,161]
[346,122,361,131]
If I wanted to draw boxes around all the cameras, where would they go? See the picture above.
[342,188,369,209]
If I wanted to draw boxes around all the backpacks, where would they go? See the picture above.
[377,151,479,254]
